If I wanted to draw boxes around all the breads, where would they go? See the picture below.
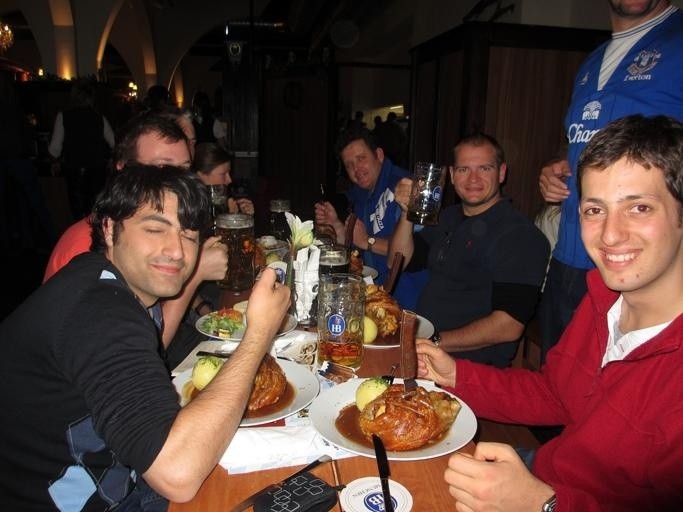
[361,385,438,451]
[248,353,287,412]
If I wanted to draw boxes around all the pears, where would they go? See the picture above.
[350,316,378,343]
[356,380,387,412]
[192,357,225,391]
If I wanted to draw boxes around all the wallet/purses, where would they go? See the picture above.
[252,469,339,511]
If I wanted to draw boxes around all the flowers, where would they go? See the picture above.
[284,209,323,257]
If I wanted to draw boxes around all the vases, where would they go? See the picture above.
[285,255,297,302]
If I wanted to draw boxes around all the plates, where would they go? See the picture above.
[168,357,319,430]
[255,237,288,251]
[194,307,297,342]
[308,377,480,462]
[363,306,437,350]
[359,266,379,281]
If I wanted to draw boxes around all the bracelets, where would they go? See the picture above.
[540,493,558,509]
[432,330,443,350]
[193,300,216,314]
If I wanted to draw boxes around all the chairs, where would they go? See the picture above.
[468,221,550,370]
[521,293,552,372]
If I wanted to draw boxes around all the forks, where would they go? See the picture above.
[381,361,398,385]
[195,348,294,362]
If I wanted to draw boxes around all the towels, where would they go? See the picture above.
[218,419,329,477]
[220,332,307,354]
[293,244,309,323]
[304,241,321,320]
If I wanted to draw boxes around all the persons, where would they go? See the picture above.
[43,115,232,373]
[528,2,681,434]
[385,132,551,372]
[412,112,681,511]
[531,196,561,291]
[0,158,296,511]
[311,130,429,315]
[340,109,406,166]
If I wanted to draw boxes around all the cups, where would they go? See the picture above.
[295,218,357,370]
[405,160,448,228]
[207,184,227,216]
[269,198,291,237]
[212,213,255,292]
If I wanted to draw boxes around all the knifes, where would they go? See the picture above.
[370,433,396,512]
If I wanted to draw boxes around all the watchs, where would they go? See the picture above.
[365,235,378,253]
